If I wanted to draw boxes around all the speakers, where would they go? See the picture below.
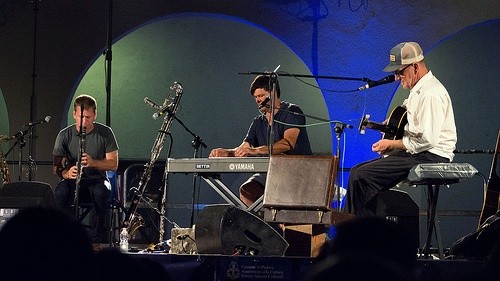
[194,204,289,257]
[373,188,421,253]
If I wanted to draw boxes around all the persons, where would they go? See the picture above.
[52,94,118,242]
[346,42,457,214]
[0,206,418,281]
[209,73,313,208]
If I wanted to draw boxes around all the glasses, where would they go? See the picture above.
[394,64,411,76]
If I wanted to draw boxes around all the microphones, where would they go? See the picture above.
[359,75,395,90]
[28,116,53,125]
[144,96,161,110]
[259,95,272,108]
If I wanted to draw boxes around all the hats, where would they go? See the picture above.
[383,41,425,73]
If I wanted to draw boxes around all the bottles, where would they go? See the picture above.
[120,228,128,253]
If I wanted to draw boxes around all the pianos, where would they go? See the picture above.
[167,153,283,250]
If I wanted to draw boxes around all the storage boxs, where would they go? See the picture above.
[264,155,354,225]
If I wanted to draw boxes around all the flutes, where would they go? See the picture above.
[81,124,88,164]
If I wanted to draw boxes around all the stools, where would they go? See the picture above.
[406,164,478,259]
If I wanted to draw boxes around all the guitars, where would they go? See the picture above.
[359,104,407,140]
